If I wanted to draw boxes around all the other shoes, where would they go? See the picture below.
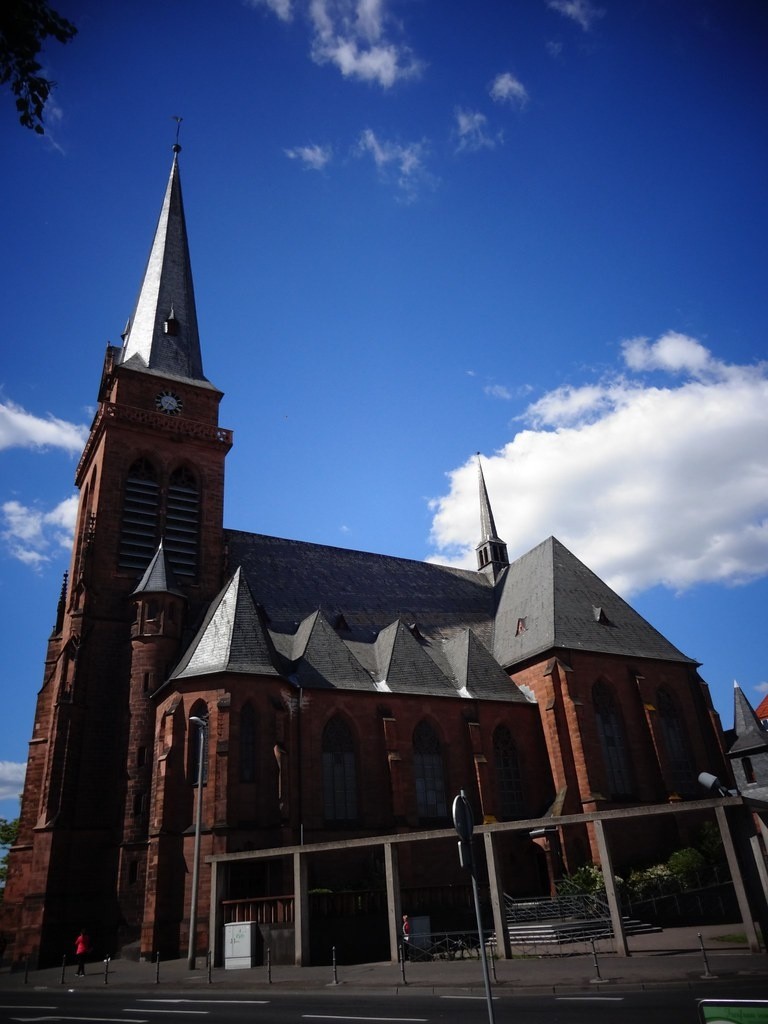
[74,974,79,977]
[79,974,85,977]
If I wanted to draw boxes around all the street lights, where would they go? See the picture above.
[185,716,207,972]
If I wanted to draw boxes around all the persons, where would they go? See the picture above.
[403,915,410,960]
[71,928,90,977]
